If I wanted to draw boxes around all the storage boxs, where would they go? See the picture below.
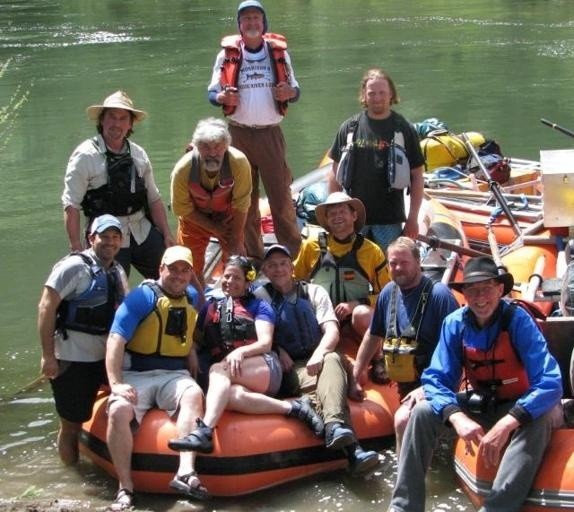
[538,147,574,229]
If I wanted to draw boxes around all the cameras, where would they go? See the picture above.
[466,387,496,415]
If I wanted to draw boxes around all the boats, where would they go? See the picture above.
[446,427,574,511]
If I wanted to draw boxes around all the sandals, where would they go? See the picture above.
[168,471,208,501]
[111,489,135,512]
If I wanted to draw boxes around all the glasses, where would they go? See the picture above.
[227,254,249,264]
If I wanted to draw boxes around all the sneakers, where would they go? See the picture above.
[371,358,390,385]
[287,396,324,437]
[167,418,215,454]
[347,448,378,475]
[325,421,354,450]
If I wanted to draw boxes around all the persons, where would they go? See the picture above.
[35,214,134,474]
[325,67,427,251]
[386,255,564,512]
[60,90,176,283]
[205,0,302,260]
[166,111,256,290]
[102,244,207,512]
[346,237,462,471]
[291,190,391,384]
[163,253,327,453]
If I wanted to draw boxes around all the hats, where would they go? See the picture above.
[161,245,194,268]
[91,214,122,236]
[263,244,291,259]
[315,192,366,233]
[448,256,514,298]
[87,90,146,122]
[237,0,265,15]
